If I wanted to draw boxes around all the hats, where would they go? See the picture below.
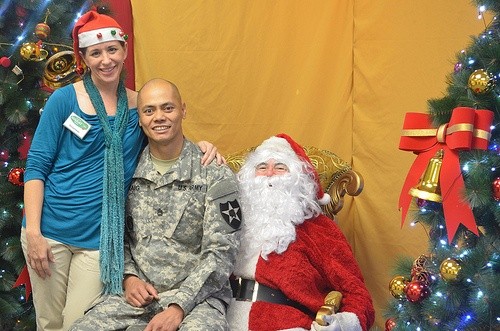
[257,133,331,205]
[72,10,128,75]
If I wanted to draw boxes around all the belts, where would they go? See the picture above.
[227,274,315,317]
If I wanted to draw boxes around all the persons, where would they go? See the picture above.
[20,11,227,330]
[68,78,243,331]
[227,133,376,331]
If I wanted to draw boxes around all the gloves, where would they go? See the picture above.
[310,312,363,331]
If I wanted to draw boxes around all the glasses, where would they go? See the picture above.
[256,162,289,172]
